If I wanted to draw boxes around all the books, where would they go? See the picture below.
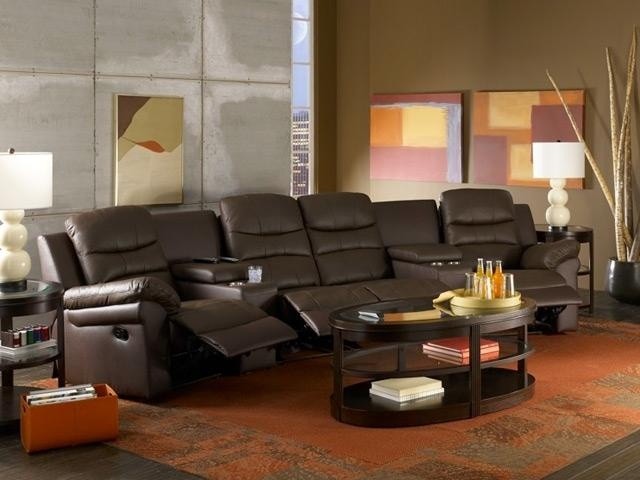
[422,348,500,364]
[421,335,499,354]
[371,399,444,412]
[358,298,443,322]
[369,394,445,407]
[0,337,61,361]
[426,354,496,366]
[26,384,99,407]
[370,377,445,398]
[369,385,445,404]
[422,341,500,359]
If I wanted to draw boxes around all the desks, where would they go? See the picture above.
[533,223,597,311]
[1,280,73,437]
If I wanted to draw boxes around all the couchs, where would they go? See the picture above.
[41,189,590,397]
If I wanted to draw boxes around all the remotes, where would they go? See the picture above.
[358,309,385,319]
[218,255,240,263]
[193,257,219,264]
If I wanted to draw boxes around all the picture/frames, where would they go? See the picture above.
[112,92,184,206]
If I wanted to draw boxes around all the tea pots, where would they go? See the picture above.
[463,271,481,297]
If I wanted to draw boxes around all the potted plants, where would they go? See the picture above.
[545,21,640,304]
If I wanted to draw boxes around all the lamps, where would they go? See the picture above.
[0,148,61,291]
[529,139,589,229]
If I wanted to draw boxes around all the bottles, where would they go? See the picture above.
[473,256,507,299]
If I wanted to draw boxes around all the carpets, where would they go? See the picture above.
[19,314,639,480]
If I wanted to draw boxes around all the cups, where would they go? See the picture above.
[503,273,515,298]
[247,265,264,284]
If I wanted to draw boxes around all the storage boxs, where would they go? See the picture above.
[18,383,125,453]
